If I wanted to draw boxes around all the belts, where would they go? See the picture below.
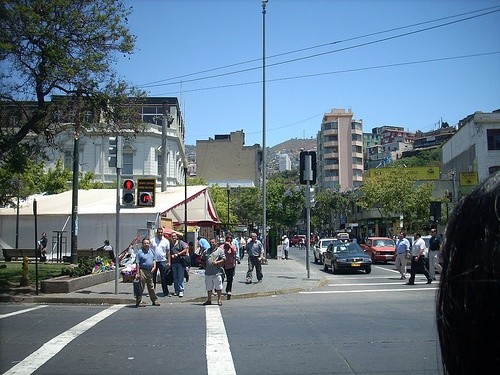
[398,253,401,254]
[140,268,148,270]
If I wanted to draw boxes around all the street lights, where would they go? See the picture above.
[182,167,197,243]
[227,185,230,230]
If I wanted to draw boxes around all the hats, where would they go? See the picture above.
[284,235,287,237]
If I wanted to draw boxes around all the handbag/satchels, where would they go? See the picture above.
[180,255,190,267]
[133,279,143,296]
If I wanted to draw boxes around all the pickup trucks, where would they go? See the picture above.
[360,237,397,264]
[289,235,315,247]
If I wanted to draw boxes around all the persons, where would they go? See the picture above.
[147,228,172,297]
[196,236,211,269]
[220,230,265,297]
[395,231,411,280]
[298,237,306,250]
[405,233,434,285]
[101,240,114,252]
[281,235,290,260]
[134,237,160,314]
[168,231,189,298]
[202,238,226,305]
[38,231,48,257]
[428,229,443,281]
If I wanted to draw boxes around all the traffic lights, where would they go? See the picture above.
[123,178,135,207]
[305,185,315,208]
[138,191,152,206]
[108,136,123,168]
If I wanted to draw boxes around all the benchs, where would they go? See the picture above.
[2,248,46,261]
[75,250,115,259]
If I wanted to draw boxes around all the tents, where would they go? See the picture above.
[0,184,220,259]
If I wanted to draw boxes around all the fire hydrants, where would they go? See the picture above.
[19,255,30,286]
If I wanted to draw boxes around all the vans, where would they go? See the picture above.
[396,235,445,267]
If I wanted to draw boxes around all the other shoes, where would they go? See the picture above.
[203,300,211,305]
[152,302,160,306]
[406,283,414,285]
[167,293,172,296]
[225,291,232,295]
[174,292,179,296]
[136,304,142,307]
[147,294,150,297]
[432,278,436,281]
[258,279,262,282]
[401,276,406,280]
[246,281,252,284]
[186,276,189,282]
[217,301,222,305]
[179,292,183,297]
[426,278,433,284]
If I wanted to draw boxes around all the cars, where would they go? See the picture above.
[313,238,349,265]
[323,233,372,274]
